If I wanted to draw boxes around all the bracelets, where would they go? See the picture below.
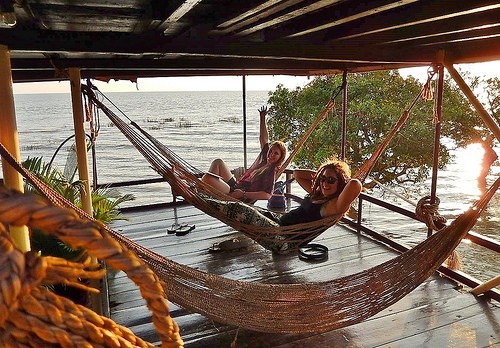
[242,191,246,200]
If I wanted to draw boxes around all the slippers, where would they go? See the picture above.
[167,222,188,234]
[176,223,195,236]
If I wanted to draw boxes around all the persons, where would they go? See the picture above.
[202,105,286,200]
[170,161,362,255]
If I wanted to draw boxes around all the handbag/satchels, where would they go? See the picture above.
[268,178,295,209]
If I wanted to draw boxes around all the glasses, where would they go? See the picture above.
[318,174,340,184]
